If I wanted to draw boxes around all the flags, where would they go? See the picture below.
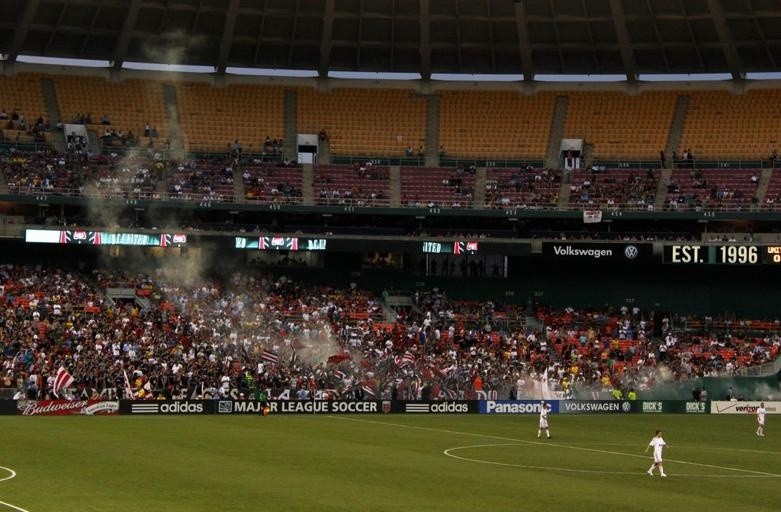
[262,405,270,416]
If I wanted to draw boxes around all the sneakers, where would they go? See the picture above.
[756,431,765,436]
[547,436,552,439]
[538,435,541,438]
[661,473,668,476]
[648,470,653,475]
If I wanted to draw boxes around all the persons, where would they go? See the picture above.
[1,108,781,241]
[643,429,670,478]
[755,402,767,438]
[2,258,781,401]
[537,403,552,440]
[257,388,267,417]
